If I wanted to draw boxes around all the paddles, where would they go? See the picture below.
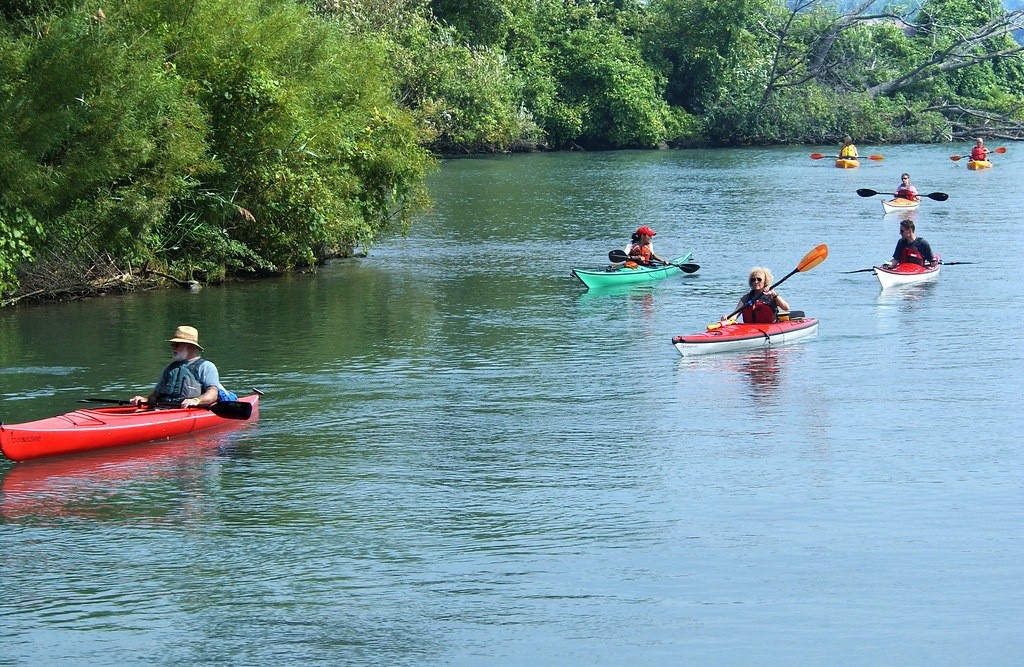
[608,249,701,274]
[605,260,639,272]
[836,260,978,275]
[725,243,830,319]
[810,152,885,163]
[950,146,1007,161]
[75,397,253,421]
[856,189,950,201]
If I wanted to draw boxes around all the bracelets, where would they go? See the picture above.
[773,293,778,299]
[193,397,201,405]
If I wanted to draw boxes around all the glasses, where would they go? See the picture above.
[751,278,763,282]
[900,229,907,233]
[902,177,907,180]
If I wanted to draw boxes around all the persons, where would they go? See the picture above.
[895,173,920,201]
[882,220,934,270]
[624,226,669,266]
[840,136,858,160]
[969,138,990,161]
[130,326,219,409]
[721,268,789,323]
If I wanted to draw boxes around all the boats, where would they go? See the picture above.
[834,158,860,170]
[672,310,818,357]
[966,160,993,170]
[0,386,264,462]
[570,253,695,289]
[881,195,922,213]
[873,250,942,289]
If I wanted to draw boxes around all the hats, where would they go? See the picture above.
[637,226,654,236]
[165,325,203,352]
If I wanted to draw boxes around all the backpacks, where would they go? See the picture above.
[158,358,207,406]
